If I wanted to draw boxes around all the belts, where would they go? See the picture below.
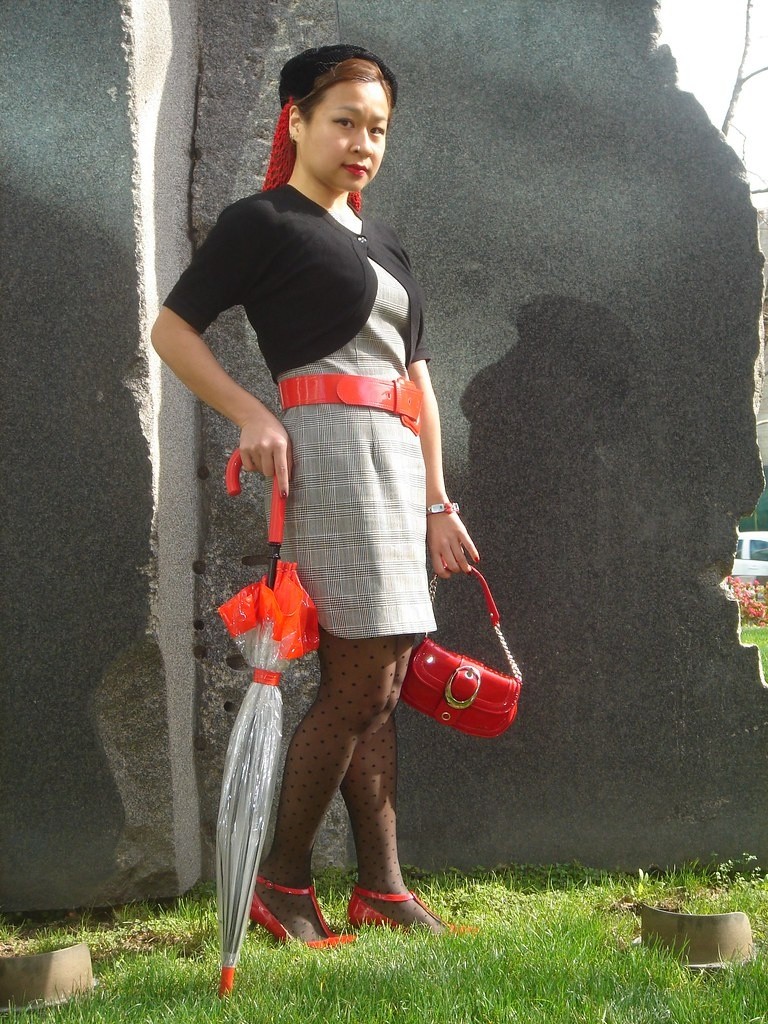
[278,375,424,434]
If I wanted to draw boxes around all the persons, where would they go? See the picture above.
[151,44,481,952]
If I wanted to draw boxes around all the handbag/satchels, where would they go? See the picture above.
[401,563,525,738]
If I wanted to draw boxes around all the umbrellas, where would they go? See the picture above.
[216,451,320,998]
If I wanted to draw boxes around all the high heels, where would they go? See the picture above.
[249,875,356,950]
[347,884,479,938]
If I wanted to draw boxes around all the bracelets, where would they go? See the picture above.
[427,502,462,515]
